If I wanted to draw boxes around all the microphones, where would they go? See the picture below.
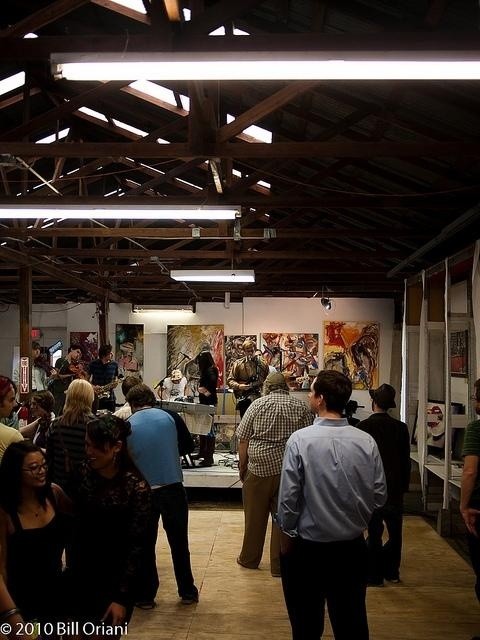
[248,350,253,361]
[181,352,191,360]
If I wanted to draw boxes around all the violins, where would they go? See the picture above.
[68,362,99,396]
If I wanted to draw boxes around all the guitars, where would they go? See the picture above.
[234,370,297,401]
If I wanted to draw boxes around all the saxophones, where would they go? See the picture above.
[95,368,126,398]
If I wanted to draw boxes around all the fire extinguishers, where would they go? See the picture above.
[16,400,31,431]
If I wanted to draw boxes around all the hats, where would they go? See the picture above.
[170,370,181,380]
[369,384,396,408]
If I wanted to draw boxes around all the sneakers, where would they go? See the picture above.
[182,586,197,603]
[366,574,400,586]
[237,556,257,568]
[271,569,281,577]
[136,600,153,609]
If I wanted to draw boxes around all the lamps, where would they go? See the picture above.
[49,50,480,81]
[170,269,256,282]
[1,205,246,219]
[321,286,334,311]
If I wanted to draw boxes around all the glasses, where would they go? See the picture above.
[23,461,48,476]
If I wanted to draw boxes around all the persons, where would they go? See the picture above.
[225,339,268,423]
[0,341,217,640]
[460,380,479,640]
[273,370,388,640]
[233,371,316,579]
[255,348,277,382]
[356,383,410,586]
